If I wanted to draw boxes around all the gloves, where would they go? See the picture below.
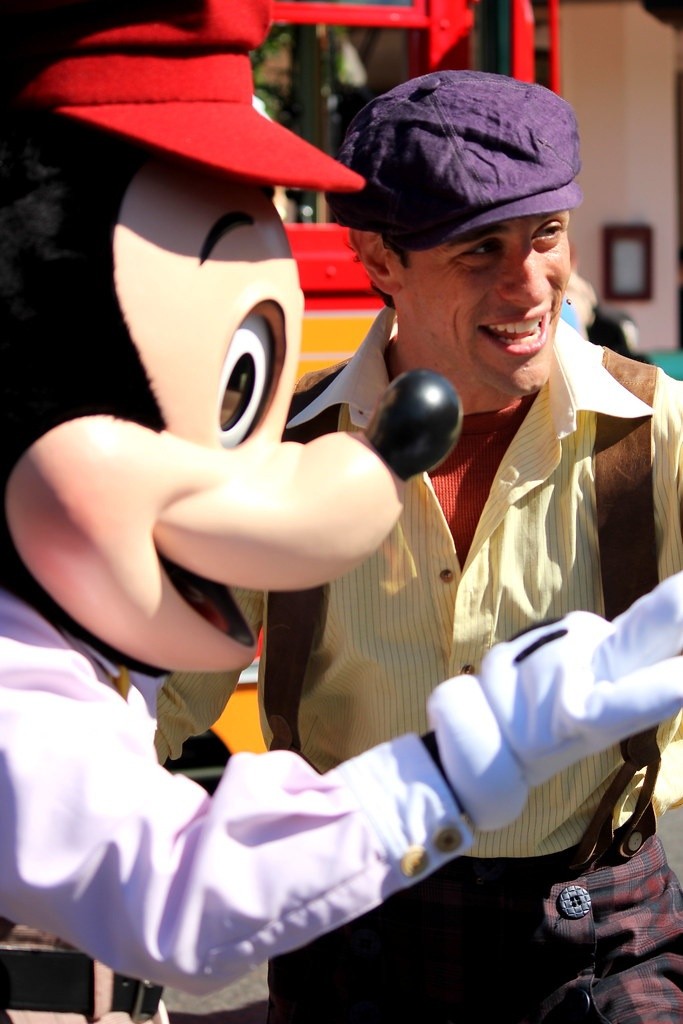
[423,561,683,832]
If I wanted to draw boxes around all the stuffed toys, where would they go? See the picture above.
[1,1,683,1024]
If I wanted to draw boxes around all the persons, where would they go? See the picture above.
[153,70,683,1024]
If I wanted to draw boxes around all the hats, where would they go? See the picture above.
[328,67,584,252]
[0,1,366,195]
